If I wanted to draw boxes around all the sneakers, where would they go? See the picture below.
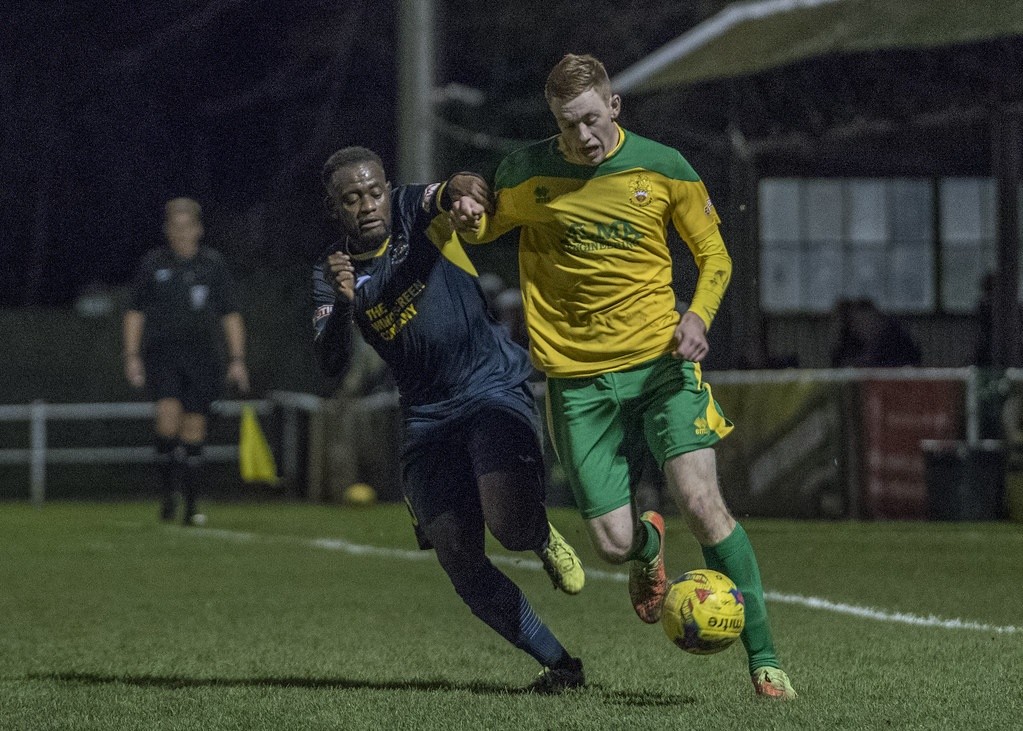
[532,521,585,594]
[627,511,667,624]
[751,665,798,701]
[514,656,585,694]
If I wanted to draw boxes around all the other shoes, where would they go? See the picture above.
[184,507,205,526]
[159,494,180,521]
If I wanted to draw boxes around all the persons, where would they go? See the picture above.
[312,146,586,693]
[448,53,799,701]
[121,197,252,527]
[818,297,923,368]
[964,273,995,366]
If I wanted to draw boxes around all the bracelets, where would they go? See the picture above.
[228,354,244,362]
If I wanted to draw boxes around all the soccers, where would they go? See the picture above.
[660,568,746,656]
